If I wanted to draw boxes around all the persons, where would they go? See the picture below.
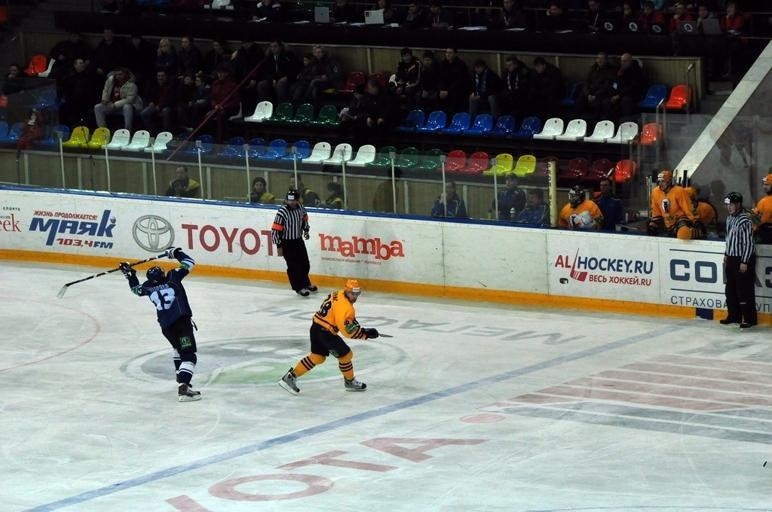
[2,2,772,246]
[280,278,379,393]
[117,244,202,401]
[270,189,319,297]
[719,191,758,330]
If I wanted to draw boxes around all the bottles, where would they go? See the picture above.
[487,208,492,220]
[510,207,516,222]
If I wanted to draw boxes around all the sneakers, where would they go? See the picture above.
[344,377,366,389]
[297,288,309,296]
[306,285,317,291]
[740,322,752,328]
[282,371,300,392]
[720,318,742,324]
[178,384,200,396]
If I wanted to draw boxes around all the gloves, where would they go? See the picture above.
[165,246,182,259]
[120,262,136,278]
[366,328,378,338]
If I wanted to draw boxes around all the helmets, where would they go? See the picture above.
[505,169,772,208]
[286,189,300,200]
[146,266,165,283]
[345,279,361,293]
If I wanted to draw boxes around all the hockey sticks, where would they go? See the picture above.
[56,253,168,301]
